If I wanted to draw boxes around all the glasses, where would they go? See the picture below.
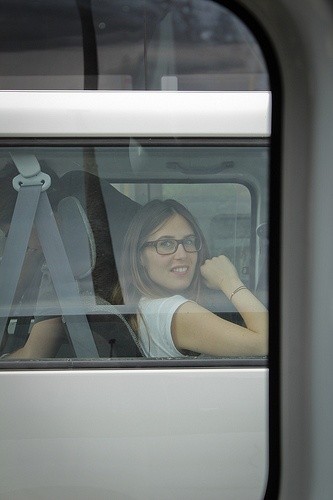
[143,235,202,256]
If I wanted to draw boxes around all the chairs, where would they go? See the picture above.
[50,168,233,361]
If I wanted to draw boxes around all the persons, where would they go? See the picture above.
[109,197,269,358]
[1,159,144,360]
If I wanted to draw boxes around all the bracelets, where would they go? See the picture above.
[230,286,246,298]
